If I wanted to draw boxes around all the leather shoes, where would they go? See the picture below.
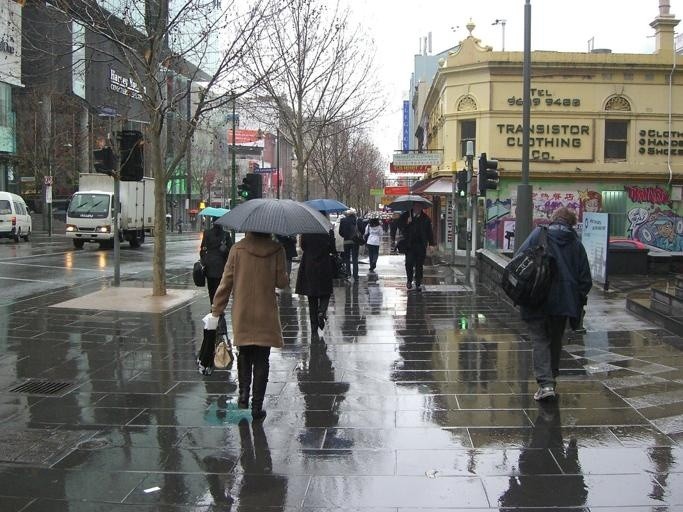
[318,312,324,329]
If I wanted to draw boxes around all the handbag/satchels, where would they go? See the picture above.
[213,341,233,369]
[397,239,407,252]
[193,262,205,286]
[502,246,551,307]
[332,251,350,279]
[353,230,365,245]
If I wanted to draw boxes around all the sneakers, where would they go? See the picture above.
[534,386,555,401]
[253,410,265,419]
[238,394,248,409]
[416,282,422,291]
[406,281,412,289]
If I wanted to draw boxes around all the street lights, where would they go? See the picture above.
[490,19,506,53]
[32,101,45,193]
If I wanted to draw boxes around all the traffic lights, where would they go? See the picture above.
[91,146,114,175]
[240,175,263,201]
[236,183,245,197]
[477,157,499,191]
[457,168,468,199]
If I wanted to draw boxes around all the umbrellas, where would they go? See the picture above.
[197,207,231,218]
[298,431,354,458]
[302,198,350,214]
[212,198,334,237]
[203,402,253,425]
[387,194,433,218]
[190,210,198,213]
[392,367,436,387]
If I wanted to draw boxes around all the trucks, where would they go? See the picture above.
[63,172,156,251]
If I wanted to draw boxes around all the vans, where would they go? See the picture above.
[0,190,34,240]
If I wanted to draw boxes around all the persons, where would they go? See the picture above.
[513,206,593,402]
[392,289,437,369]
[295,209,336,335]
[296,330,349,435]
[333,201,438,291]
[237,417,288,511]
[203,317,237,407]
[580,191,602,224]
[211,231,290,417]
[200,216,233,317]
[647,216,683,252]
[341,277,367,341]
[499,395,588,511]
[276,233,297,285]
[165,214,206,234]
[278,288,300,345]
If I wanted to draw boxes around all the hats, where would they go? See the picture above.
[553,207,577,225]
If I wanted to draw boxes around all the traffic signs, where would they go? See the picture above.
[254,168,276,173]
